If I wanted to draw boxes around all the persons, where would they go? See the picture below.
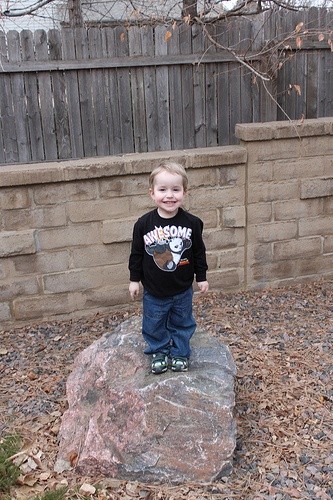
[127,162,209,375]
